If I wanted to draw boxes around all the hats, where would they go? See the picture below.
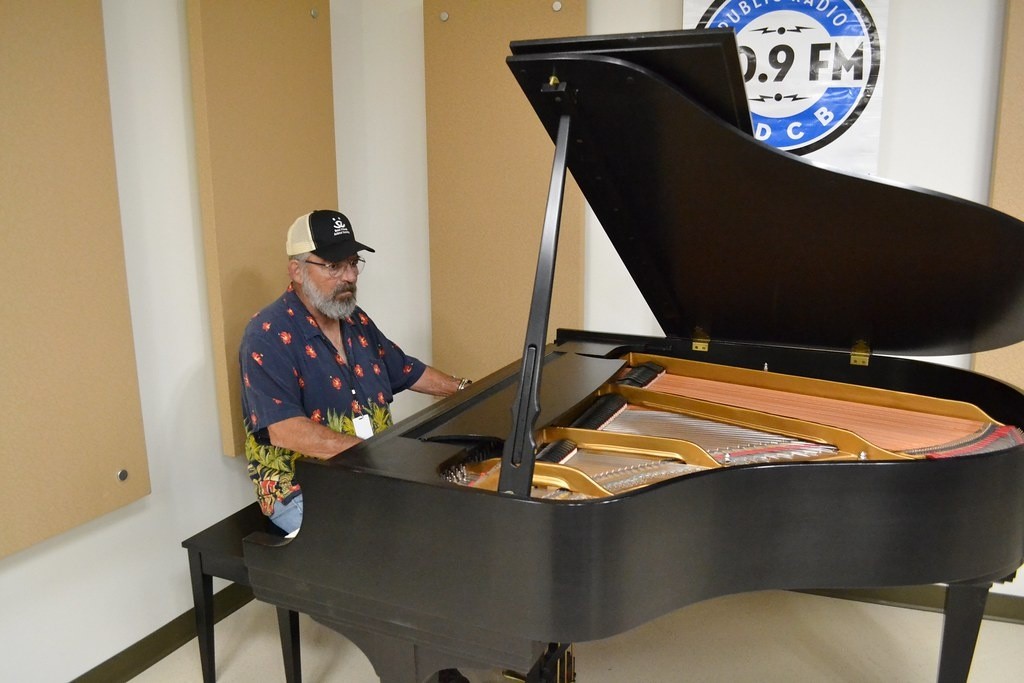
[286,209,375,262]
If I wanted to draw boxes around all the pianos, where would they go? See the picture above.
[240,26,1023,682]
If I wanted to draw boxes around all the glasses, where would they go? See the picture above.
[301,258,365,277]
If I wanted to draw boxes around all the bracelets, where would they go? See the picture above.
[458,377,472,390]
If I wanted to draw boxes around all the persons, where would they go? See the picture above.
[238,210,472,682]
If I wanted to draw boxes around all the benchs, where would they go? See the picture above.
[179,501,301,683]
